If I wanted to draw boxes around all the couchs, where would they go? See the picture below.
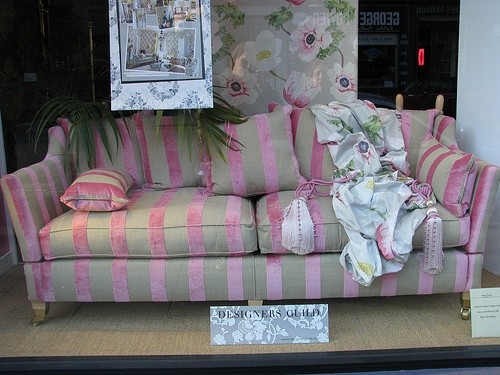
[0,93,500,328]
